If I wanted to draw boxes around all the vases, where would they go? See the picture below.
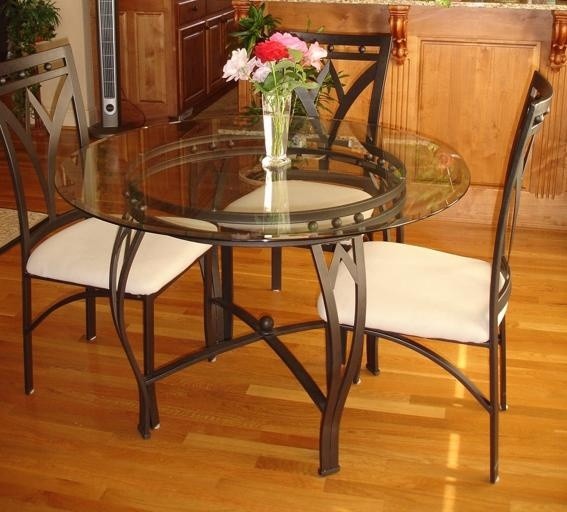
[261,93,292,167]
[263,167,291,240]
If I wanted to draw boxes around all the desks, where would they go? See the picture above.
[52,112,471,478]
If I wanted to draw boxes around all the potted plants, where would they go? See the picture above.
[225,0,352,198]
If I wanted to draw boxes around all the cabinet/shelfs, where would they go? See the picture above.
[115,0,235,129]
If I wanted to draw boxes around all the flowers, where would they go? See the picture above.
[221,31,329,156]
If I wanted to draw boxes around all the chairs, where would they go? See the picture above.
[269,27,394,292]
[315,69,555,485]
[0,43,217,432]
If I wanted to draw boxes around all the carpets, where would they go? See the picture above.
[0,207,49,252]
